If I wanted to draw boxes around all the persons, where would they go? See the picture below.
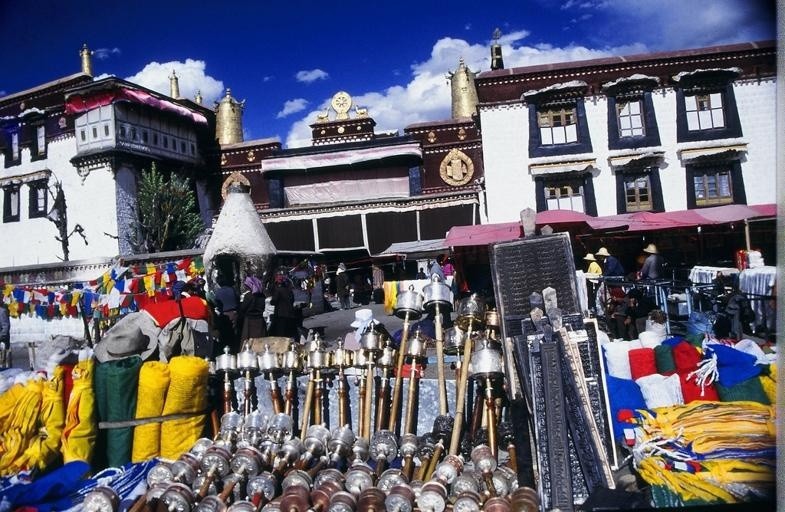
[178,254,436,355]
[0,301,12,352]
[427,254,448,282]
[581,238,677,340]
[442,259,454,277]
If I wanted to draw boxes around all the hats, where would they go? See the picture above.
[595,247,611,256]
[350,309,380,328]
[94,322,158,364]
[583,253,597,261]
[643,244,656,254]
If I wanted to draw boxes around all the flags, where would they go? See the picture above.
[0,254,205,330]
